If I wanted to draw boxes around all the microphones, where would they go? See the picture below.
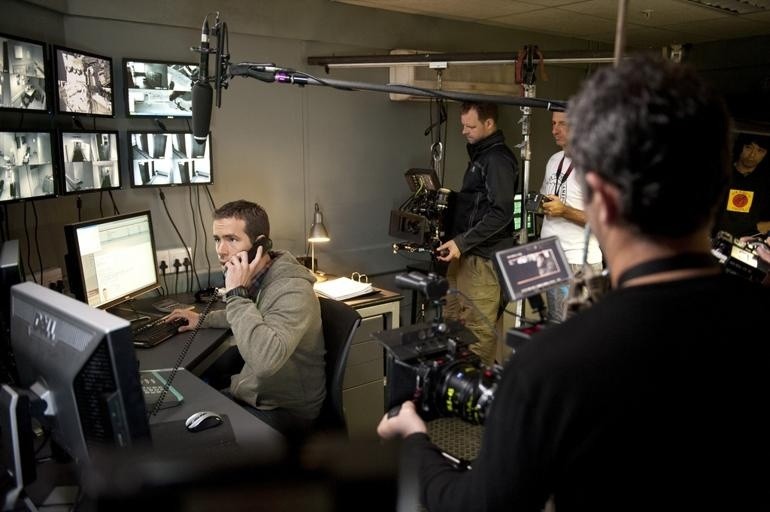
[192,23,213,144]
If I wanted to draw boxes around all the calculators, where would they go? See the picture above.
[151,298,196,312]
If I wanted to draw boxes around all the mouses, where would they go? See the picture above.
[185,411,223,432]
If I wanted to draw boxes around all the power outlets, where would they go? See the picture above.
[156,246,193,276]
[25,266,63,293]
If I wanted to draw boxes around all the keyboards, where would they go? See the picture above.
[138,370,184,410]
[131,315,188,348]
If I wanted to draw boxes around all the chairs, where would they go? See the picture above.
[296,296,365,442]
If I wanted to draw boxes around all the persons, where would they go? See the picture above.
[434,95,518,364]
[373,53,770,508]
[535,103,604,277]
[164,199,326,429]
[718,132,770,242]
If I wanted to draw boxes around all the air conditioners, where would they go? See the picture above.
[388,45,536,106]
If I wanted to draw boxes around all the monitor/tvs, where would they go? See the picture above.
[57,130,121,197]
[0,239,25,320]
[122,57,199,119]
[0,129,58,205]
[127,130,213,188]
[0,32,51,114]
[51,44,115,118]
[495,236,569,301]
[65,209,160,310]
[513,191,535,237]
[0,280,150,512]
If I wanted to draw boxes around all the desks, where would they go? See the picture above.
[1,266,407,509]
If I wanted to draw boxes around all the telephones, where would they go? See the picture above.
[223,235,273,281]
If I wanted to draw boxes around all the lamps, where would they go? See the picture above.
[305,201,332,270]
